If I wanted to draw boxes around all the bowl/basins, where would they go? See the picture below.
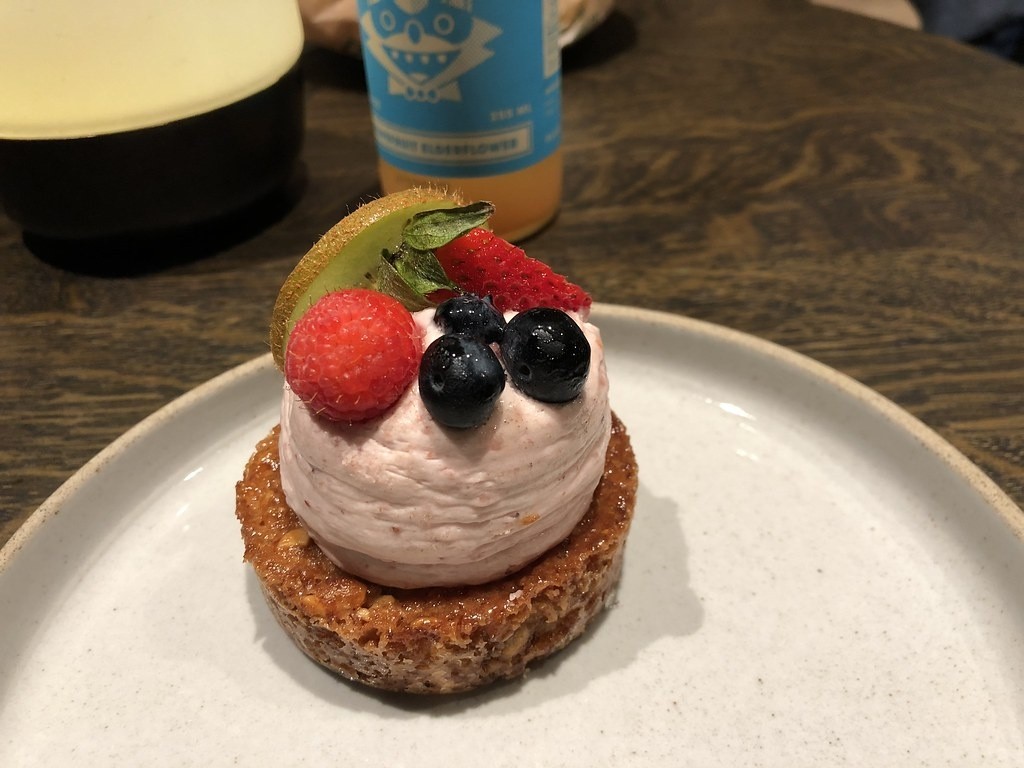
[0,0,307,235]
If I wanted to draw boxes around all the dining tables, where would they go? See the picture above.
[0,1,1024,545]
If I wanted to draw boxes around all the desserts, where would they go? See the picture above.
[234,187,640,695]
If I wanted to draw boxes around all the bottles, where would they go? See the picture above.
[357,0,562,243]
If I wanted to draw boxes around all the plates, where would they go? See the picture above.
[0,301,1024,768]
